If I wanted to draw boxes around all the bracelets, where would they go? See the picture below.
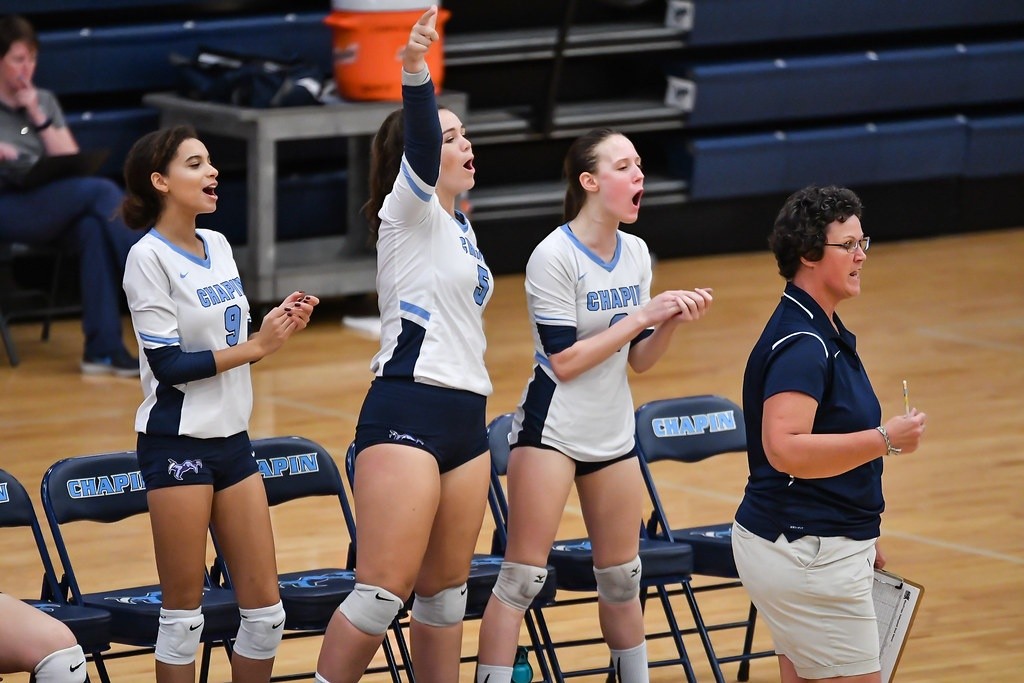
[875,425,901,457]
[33,116,53,133]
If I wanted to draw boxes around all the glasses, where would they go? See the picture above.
[823,236,870,253]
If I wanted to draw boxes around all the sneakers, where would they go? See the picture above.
[81,347,140,377]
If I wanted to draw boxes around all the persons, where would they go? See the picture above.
[117,124,320,683]
[0,592,89,683]
[475,125,712,682]
[730,184,925,683]
[311,5,494,683]
[0,13,150,377]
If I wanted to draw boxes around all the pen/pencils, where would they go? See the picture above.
[902,379,910,417]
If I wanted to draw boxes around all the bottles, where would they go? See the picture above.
[509,646,533,683]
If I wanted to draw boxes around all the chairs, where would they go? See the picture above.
[0,242,86,367]
[0,395,775,683]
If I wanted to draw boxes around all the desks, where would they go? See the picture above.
[143,92,468,303]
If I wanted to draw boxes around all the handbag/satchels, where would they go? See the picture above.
[180,46,326,108]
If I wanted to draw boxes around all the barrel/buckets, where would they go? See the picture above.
[323,0,451,102]
